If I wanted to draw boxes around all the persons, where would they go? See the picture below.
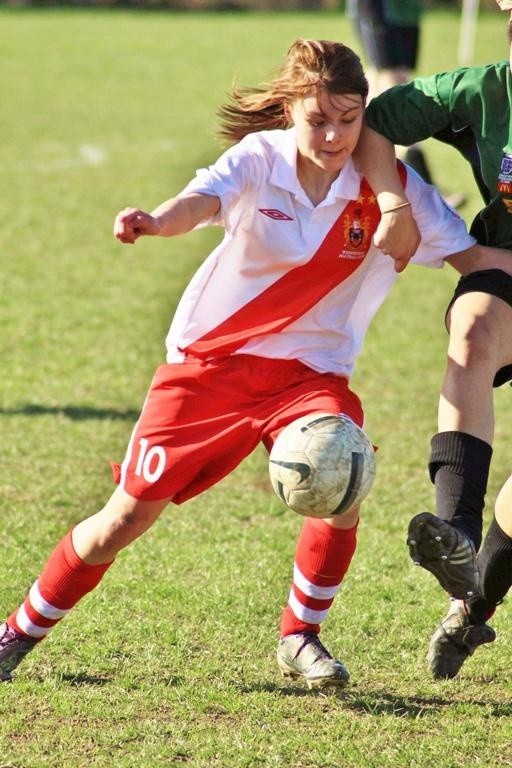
[0,39,512,681]
[348,0,467,209]
[349,16,512,682]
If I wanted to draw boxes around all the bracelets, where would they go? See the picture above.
[381,202,412,214]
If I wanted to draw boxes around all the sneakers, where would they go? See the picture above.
[276,631,350,691]
[0,620,37,680]
[407,512,496,683]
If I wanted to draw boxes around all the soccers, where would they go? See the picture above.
[268,411,375,517]
